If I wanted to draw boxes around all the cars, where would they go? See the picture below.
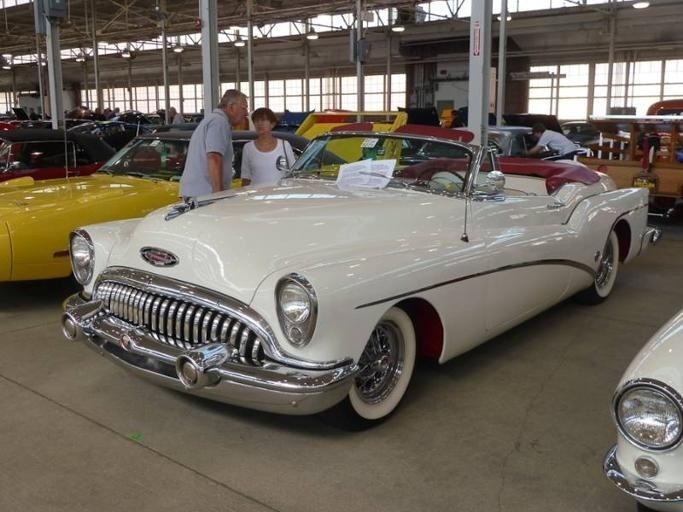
[603,307,683,512]
[0,106,204,182]
[0,124,348,283]
[60,123,663,432]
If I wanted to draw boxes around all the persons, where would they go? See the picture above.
[169,106,184,125]
[178,89,249,204]
[240,108,296,188]
[527,122,577,161]
[30,107,120,120]
